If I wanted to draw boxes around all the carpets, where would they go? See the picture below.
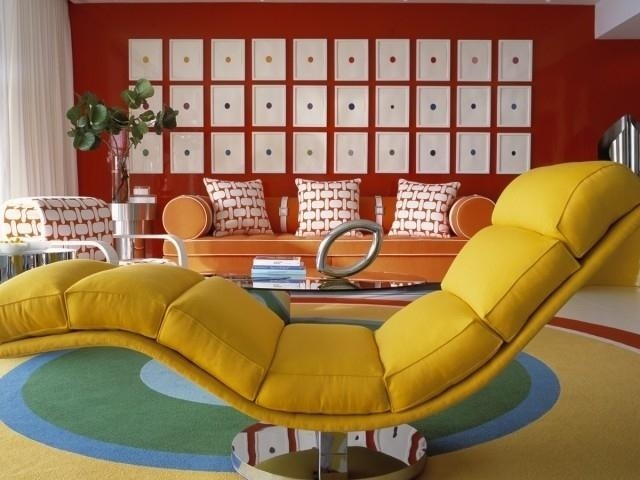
[0,347,563,470]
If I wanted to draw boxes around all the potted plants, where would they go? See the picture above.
[66,78,179,203]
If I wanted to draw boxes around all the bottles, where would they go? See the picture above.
[30,235,49,250]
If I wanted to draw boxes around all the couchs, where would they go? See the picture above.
[162,196,497,282]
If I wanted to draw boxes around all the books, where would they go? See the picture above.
[252,279,305,290]
[250,255,306,277]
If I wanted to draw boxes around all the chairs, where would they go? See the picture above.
[1,195,190,268]
[0,161,640,480]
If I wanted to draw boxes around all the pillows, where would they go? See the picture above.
[294,176,364,239]
[387,177,462,239]
[202,176,276,237]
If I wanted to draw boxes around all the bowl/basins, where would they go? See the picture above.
[0,242,29,254]
